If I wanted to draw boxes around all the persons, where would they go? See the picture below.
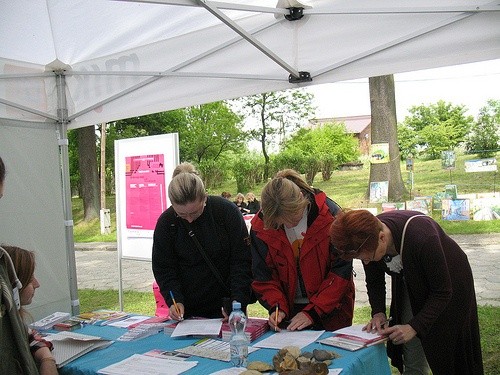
[250,169,355,331]
[329,210,484,375]
[221,191,262,216]
[152,161,256,322]
[0,156,40,375]
[0,245,58,375]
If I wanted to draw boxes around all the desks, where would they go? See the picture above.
[37,309,391,375]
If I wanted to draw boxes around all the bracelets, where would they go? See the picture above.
[39,356,57,363]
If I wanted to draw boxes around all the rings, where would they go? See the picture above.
[393,338,397,342]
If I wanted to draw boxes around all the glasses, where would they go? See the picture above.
[334,236,369,257]
[174,201,203,218]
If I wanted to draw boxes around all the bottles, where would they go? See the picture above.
[229,303,251,367]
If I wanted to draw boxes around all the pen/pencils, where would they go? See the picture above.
[373,317,393,328]
[275,306,278,331]
[169,291,183,321]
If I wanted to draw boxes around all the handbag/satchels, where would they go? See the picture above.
[222,278,257,303]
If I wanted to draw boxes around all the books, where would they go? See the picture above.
[164,317,270,344]
[53,319,81,331]
